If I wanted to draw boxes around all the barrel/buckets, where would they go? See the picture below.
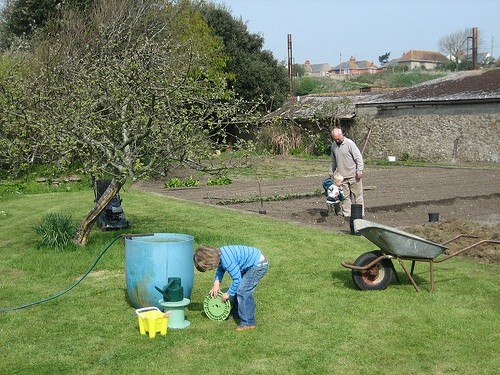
[428,213,439,221]
[350,203,362,234]
[125,233,194,312]
[388,156,395,161]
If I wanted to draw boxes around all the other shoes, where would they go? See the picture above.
[235,326,256,331]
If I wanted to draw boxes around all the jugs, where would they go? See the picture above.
[154,277,184,302]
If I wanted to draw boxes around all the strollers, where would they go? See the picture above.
[92,178,130,232]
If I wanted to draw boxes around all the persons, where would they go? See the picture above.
[323,173,345,217]
[329,128,364,219]
[193,245,269,330]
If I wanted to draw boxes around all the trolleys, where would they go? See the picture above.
[340,218,500,293]
